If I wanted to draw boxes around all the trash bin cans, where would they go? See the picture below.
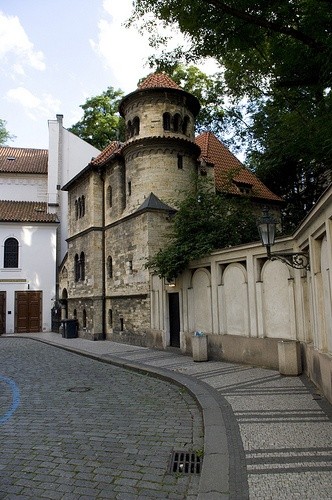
[277,339,303,376]
[60,319,66,338]
[191,331,209,362]
[64,319,79,338]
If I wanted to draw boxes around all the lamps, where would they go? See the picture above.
[256,206,310,271]
